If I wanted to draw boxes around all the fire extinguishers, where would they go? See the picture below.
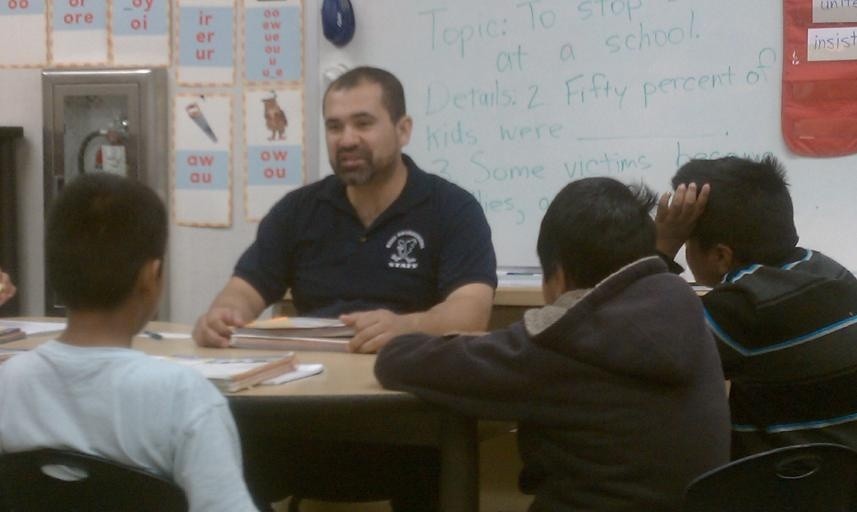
[78,116,134,179]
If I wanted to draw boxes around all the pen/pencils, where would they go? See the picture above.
[144,330,162,339]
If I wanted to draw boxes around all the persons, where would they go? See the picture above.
[649,152,857,473]
[373,175,734,510]
[190,63,499,510]
[0,169,262,510]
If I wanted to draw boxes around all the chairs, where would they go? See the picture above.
[0,448,188,512]
[684,443,856,511]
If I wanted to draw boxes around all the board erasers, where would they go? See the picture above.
[322,0,355,47]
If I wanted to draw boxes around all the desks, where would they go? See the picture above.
[0,315,481,512]
[271,274,711,331]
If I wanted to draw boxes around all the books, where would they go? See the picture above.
[0,331,25,344]
[0,326,20,337]
[0,347,29,361]
[232,314,357,339]
[227,332,354,354]
[156,350,300,395]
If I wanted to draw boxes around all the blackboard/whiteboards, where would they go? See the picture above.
[322,0,783,269]
